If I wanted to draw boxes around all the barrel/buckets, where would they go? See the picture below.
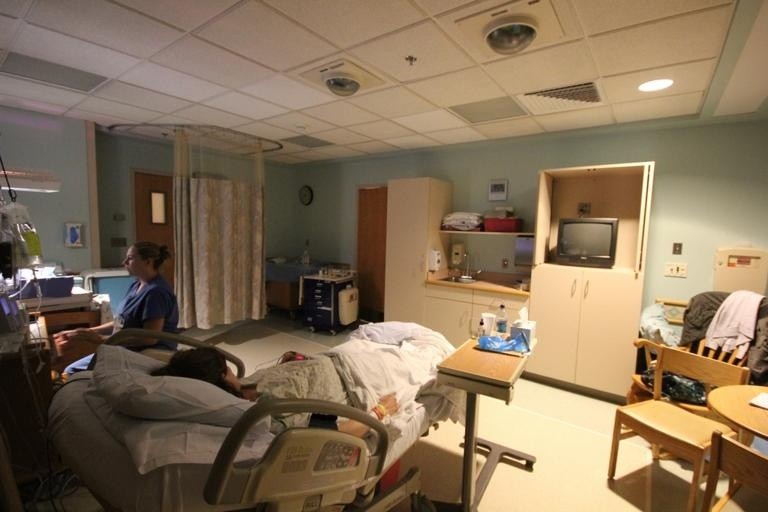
[296,238,316,272]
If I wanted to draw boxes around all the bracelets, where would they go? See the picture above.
[371,404,387,420]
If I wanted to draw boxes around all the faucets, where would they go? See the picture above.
[464,248,472,276]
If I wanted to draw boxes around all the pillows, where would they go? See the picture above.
[93,343,271,433]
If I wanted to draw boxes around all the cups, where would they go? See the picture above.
[470,317,482,339]
[519,279,530,292]
[481,312,495,337]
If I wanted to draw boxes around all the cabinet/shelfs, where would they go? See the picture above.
[424,281,530,349]
[525,161,655,406]
[298,272,358,338]
[384,176,454,321]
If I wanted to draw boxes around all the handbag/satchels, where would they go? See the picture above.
[641,359,715,403]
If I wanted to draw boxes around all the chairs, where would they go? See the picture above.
[608,291,768,512]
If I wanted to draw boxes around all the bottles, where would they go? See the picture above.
[495,304,508,341]
[476,319,484,343]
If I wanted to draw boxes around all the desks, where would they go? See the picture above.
[0,316,50,352]
[436,336,538,512]
[707,385,768,442]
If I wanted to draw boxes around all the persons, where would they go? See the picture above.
[51,240,180,377]
[150,346,401,439]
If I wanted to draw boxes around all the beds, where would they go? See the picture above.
[48,320,458,511]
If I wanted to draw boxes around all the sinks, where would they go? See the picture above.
[437,276,477,283]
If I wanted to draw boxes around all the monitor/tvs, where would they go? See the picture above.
[555,218,618,268]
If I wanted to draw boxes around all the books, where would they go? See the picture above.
[748,390,768,410]
